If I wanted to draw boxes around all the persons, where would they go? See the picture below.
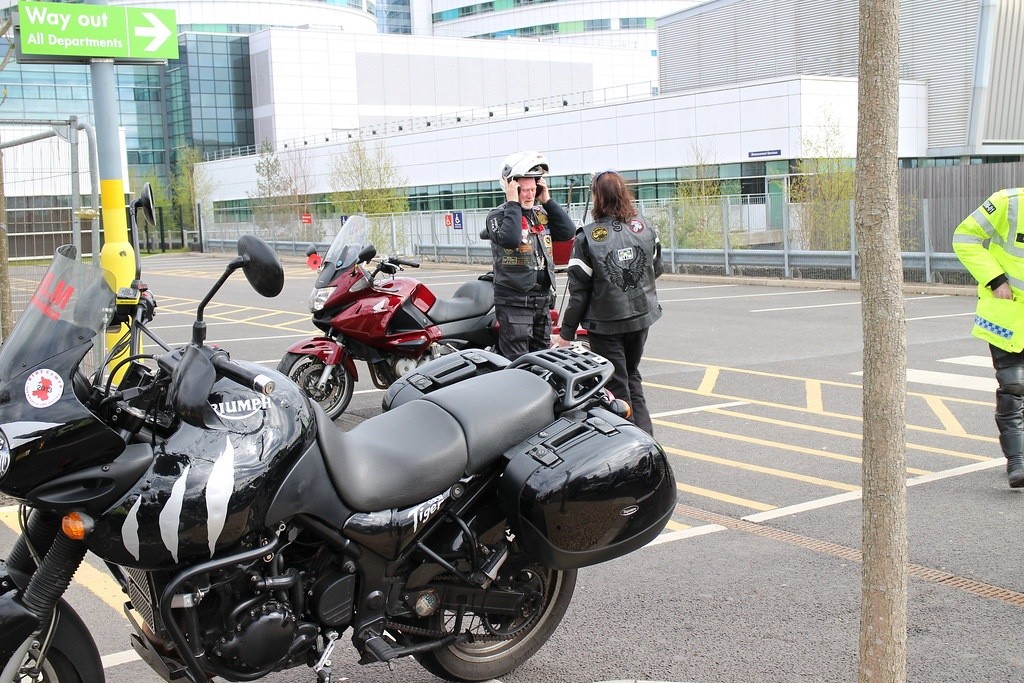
[555,170,664,442]
[485,147,579,359]
[951,185,1024,488]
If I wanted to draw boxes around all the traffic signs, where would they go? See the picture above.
[18,0,180,60]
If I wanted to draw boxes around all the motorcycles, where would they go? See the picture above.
[0,234,680,683]
[275,214,589,423]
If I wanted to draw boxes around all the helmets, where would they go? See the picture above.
[499,151,549,195]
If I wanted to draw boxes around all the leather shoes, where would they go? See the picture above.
[1003,453,1024,486]
